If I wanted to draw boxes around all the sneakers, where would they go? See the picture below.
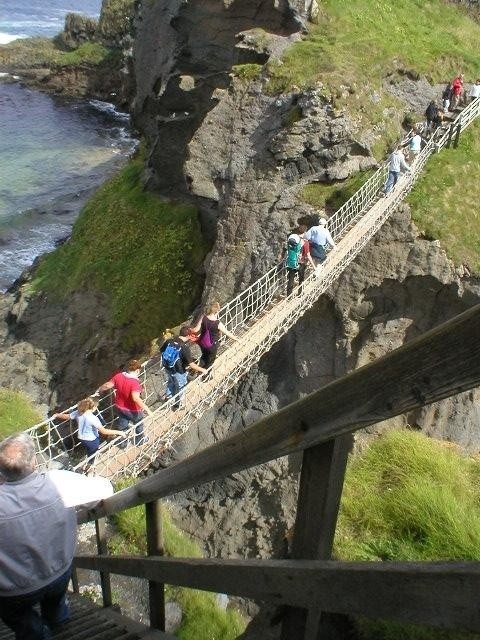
[120,438,149,449]
[173,406,183,411]
[202,375,213,380]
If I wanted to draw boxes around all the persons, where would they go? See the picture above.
[303,217,336,279]
[443,75,480,114]
[98,360,154,448]
[426,99,437,136]
[54,397,128,470]
[433,109,443,142]
[403,130,422,166]
[159,326,207,412]
[386,145,411,195]
[192,301,240,382]
[286,224,316,300]
[0,432,113,639]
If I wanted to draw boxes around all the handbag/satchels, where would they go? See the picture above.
[310,245,325,258]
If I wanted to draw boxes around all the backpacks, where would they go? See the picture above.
[162,340,181,368]
[287,239,304,270]
[198,320,216,349]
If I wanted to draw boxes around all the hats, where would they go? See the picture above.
[287,233,300,247]
[319,218,328,224]
